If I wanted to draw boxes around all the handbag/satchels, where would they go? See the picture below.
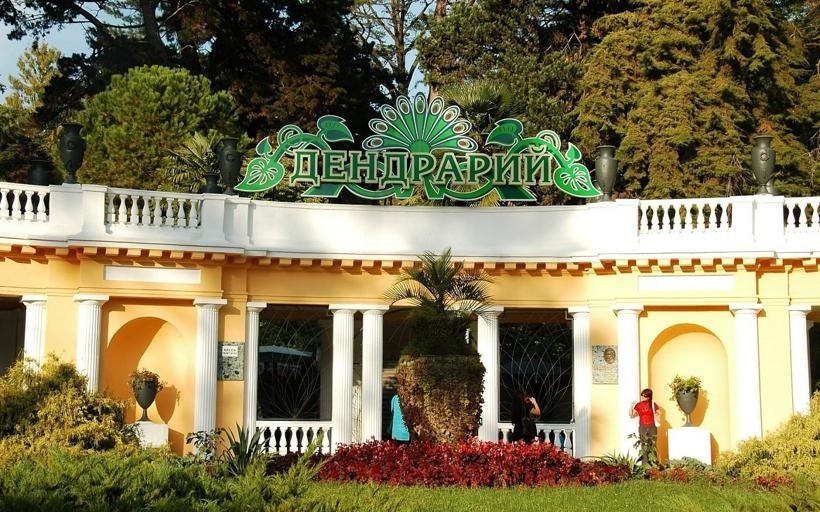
[653,412,663,428]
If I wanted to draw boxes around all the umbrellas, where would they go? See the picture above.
[258,343,316,419]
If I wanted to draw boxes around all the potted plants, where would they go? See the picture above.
[125,364,170,424]
[666,372,706,429]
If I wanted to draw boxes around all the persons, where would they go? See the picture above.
[390,394,411,445]
[629,388,663,467]
[510,391,541,445]
